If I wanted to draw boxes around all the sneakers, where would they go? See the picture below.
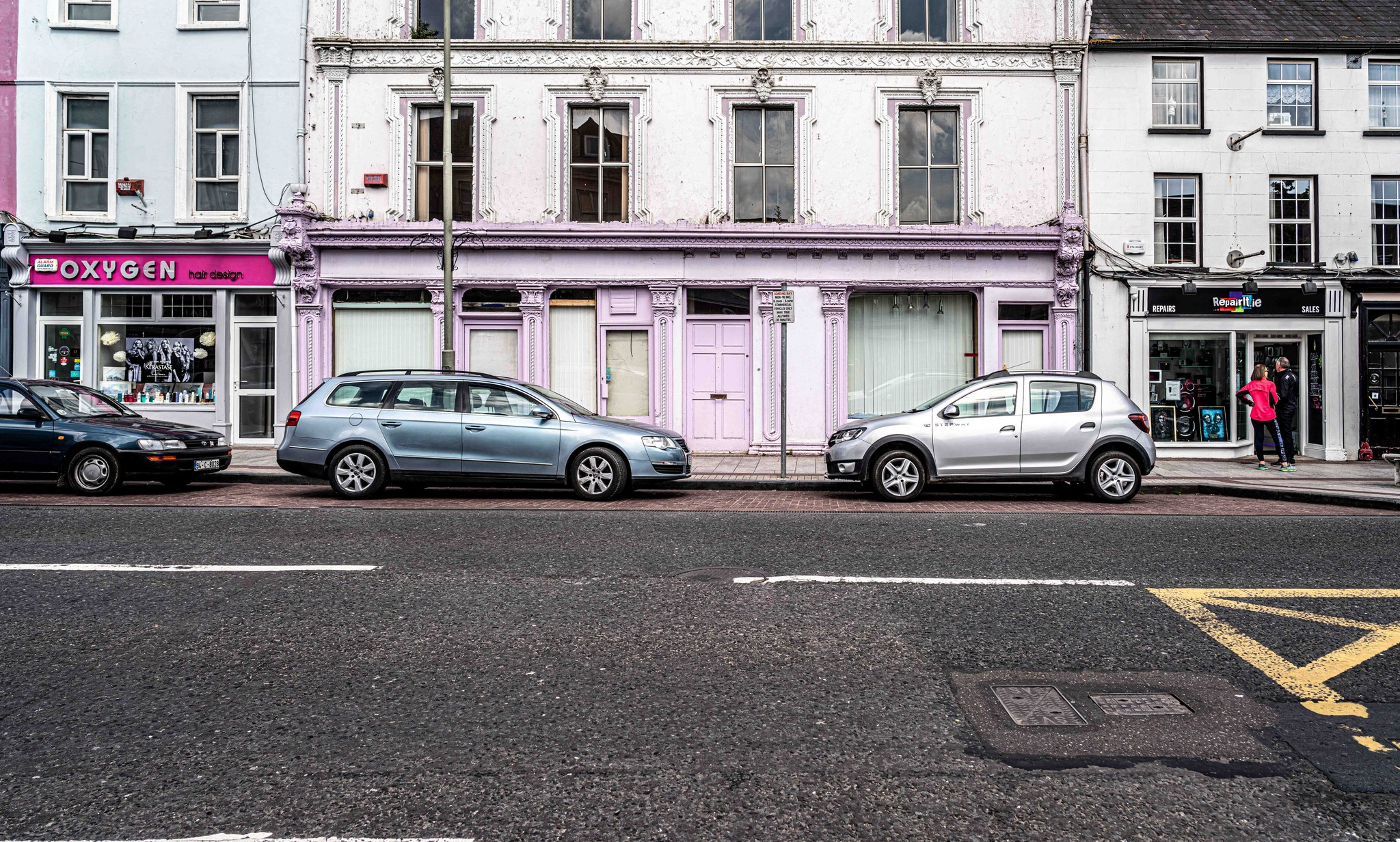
[1257,462,1269,470]
[1279,462,1297,472]
[1270,458,1289,467]
[1288,458,1296,465]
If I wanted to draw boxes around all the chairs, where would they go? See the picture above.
[485,393,499,413]
[472,393,482,408]
[987,396,1009,415]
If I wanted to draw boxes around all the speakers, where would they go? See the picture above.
[1170,381,1197,441]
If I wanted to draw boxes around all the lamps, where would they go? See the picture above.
[48,222,88,243]
[1286,274,1318,294]
[194,223,230,238]
[1233,275,1257,294]
[117,222,155,238]
[1175,274,1199,296]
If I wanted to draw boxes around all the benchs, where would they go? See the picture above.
[394,399,427,410]
[1057,391,1086,411]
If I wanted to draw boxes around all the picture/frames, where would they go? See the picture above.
[1198,406,1228,442]
[1150,405,1177,442]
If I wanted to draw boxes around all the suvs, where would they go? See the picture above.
[278,365,694,503]
[821,360,1160,503]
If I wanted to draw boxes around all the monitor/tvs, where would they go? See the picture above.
[1158,362,1172,372]
[1149,370,1162,383]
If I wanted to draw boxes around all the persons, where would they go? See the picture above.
[143,338,159,382]
[181,345,194,382]
[171,341,182,382]
[125,339,147,383]
[157,338,172,382]
[1235,364,1297,472]
[1270,357,1299,467]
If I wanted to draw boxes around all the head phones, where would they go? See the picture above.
[1200,341,1205,347]
[1159,343,1163,349]
[1184,341,1188,347]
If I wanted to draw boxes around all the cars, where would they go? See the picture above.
[0,365,233,496]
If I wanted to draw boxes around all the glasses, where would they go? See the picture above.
[1264,370,1269,374]
[1279,357,1286,366]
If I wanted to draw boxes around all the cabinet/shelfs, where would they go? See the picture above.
[1149,348,1215,386]
